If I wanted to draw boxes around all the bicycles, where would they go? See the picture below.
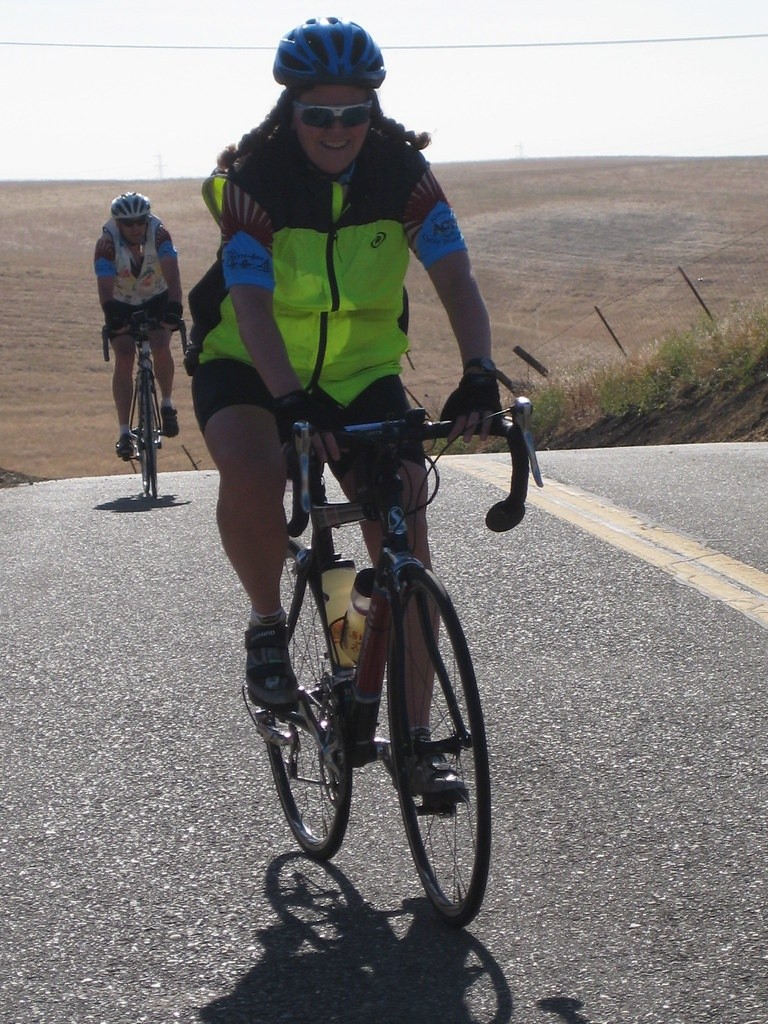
[100,308,191,499]
[238,397,546,933]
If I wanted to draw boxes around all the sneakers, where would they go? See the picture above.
[160,407,180,437]
[115,434,134,459]
[411,734,465,793]
[244,626,301,713]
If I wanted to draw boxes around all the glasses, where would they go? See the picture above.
[290,98,375,128]
[120,218,147,225]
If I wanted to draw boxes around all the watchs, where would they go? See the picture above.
[465,357,515,392]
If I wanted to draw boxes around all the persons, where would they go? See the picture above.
[93,192,183,456]
[185,16,500,795]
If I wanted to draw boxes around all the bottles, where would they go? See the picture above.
[339,568,376,663]
[320,551,361,668]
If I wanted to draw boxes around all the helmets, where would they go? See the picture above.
[111,191,150,218]
[271,16,387,91]
